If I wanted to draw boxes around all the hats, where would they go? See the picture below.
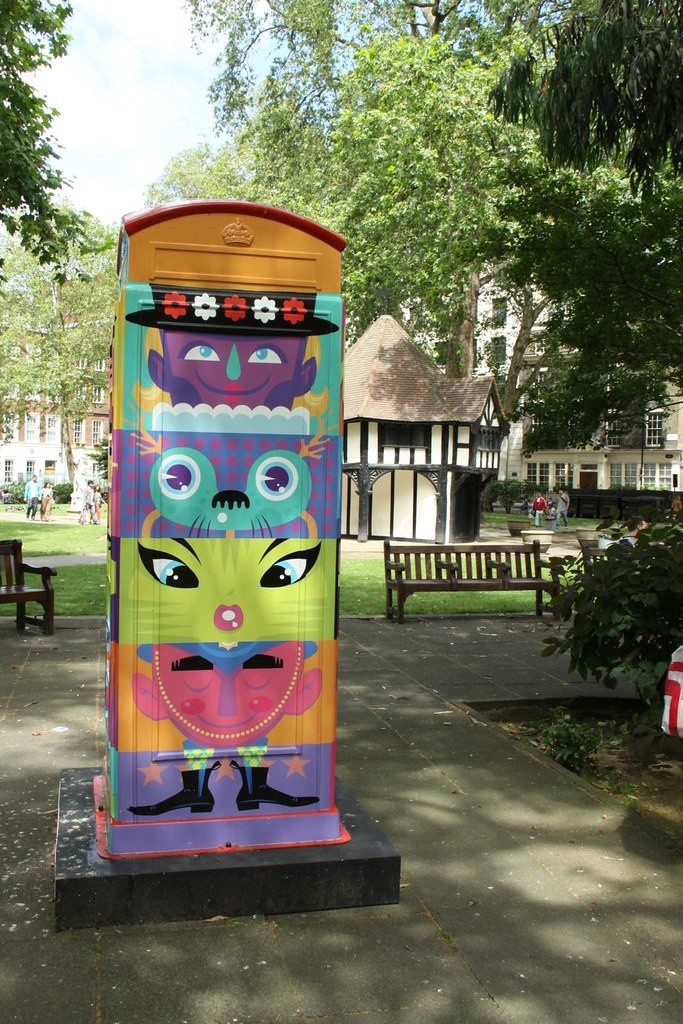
[558,488,565,491]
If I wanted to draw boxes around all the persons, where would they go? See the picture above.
[522,498,531,514]
[622,516,665,572]
[24,475,53,521]
[2,486,13,504]
[78,480,102,524]
[532,488,569,528]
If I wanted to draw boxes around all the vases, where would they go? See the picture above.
[507,520,531,536]
[575,529,607,547]
[522,530,554,553]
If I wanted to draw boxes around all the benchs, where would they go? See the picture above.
[378,540,562,624]
[579,540,607,574]
[0,539,58,635]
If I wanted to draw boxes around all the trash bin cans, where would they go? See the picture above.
[545,517,557,531]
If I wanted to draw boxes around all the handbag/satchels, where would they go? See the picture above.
[81,508,91,525]
[529,514,534,519]
